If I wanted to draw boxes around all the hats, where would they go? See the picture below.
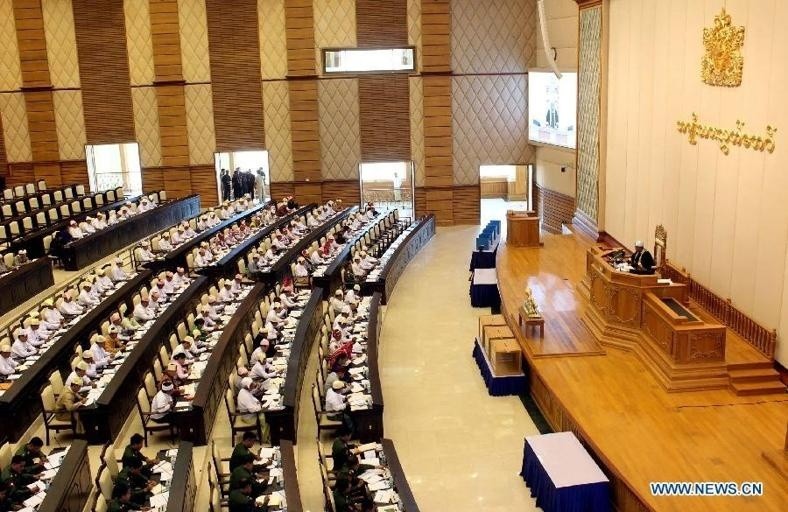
[635,240,644,247]
[140,198,267,253]
[332,284,360,391]
[1,250,124,359]
[252,200,375,263]
[69,194,154,228]
[71,266,184,387]
[168,275,292,387]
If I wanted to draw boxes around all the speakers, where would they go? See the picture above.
[561,167,565,172]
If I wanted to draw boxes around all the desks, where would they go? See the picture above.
[525,431,611,511]
[468,232,501,271]
[518,308,545,338]
[472,336,528,396]
[471,268,502,306]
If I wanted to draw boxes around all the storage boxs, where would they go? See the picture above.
[477,315,523,374]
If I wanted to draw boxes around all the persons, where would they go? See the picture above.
[0,166,382,512]
[631,241,655,272]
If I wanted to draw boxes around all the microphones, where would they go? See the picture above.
[614,251,624,259]
